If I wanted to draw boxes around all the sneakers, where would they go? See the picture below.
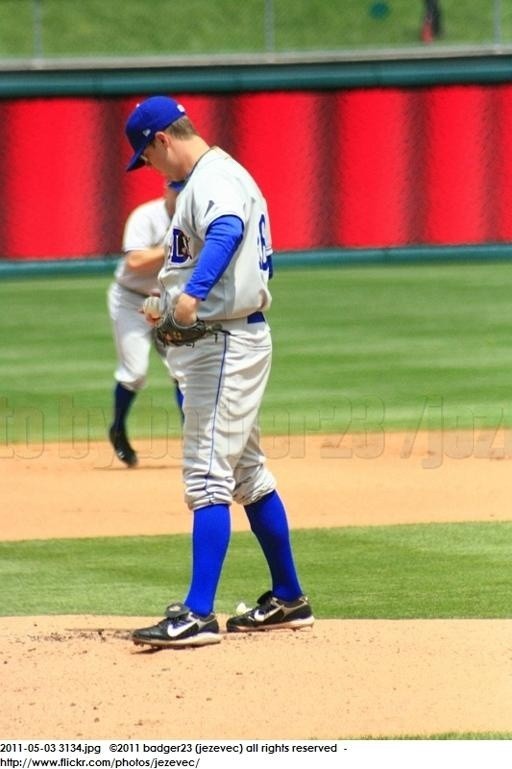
[132,603,221,645]
[110,426,136,465]
[227,591,314,631]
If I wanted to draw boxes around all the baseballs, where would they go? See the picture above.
[143,295,162,321]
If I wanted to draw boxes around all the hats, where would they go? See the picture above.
[125,96,186,171]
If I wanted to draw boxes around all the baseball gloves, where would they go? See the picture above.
[155,306,230,345]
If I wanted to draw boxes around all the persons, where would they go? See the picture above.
[105,175,184,467]
[125,95,316,647]
[419,0,446,42]
